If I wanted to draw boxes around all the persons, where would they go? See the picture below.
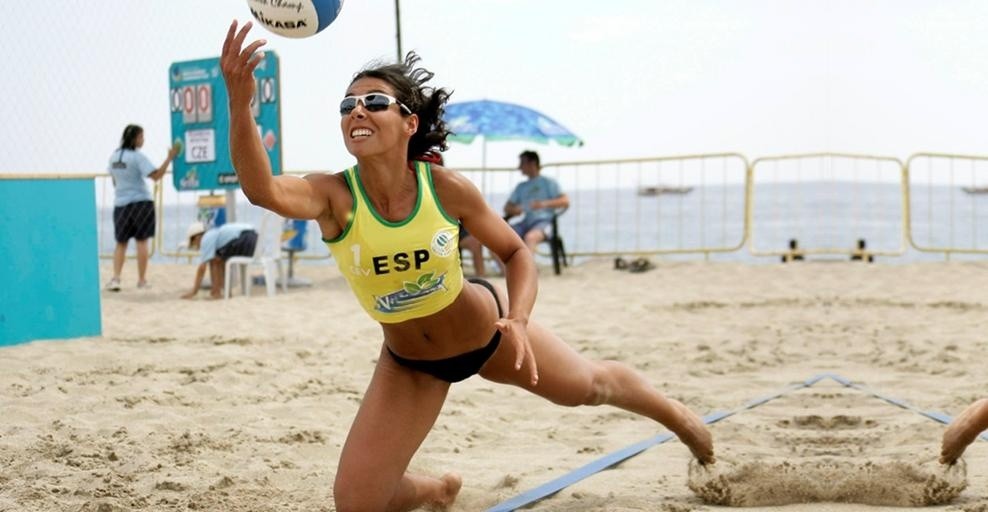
[220,19,716,511]
[106,125,179,292]
[419,150,485,280]
[180,223,259,300]
[939,398,988,467]
[490,151,569,269]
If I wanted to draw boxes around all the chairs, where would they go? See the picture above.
[498,203,569,278]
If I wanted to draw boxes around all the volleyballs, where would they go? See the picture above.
[248,0,341,39]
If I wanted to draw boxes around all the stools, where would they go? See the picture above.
[224,254,290,299]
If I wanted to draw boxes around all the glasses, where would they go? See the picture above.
[340,93,412,115]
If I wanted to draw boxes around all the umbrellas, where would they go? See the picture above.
[433,99,580,197]
